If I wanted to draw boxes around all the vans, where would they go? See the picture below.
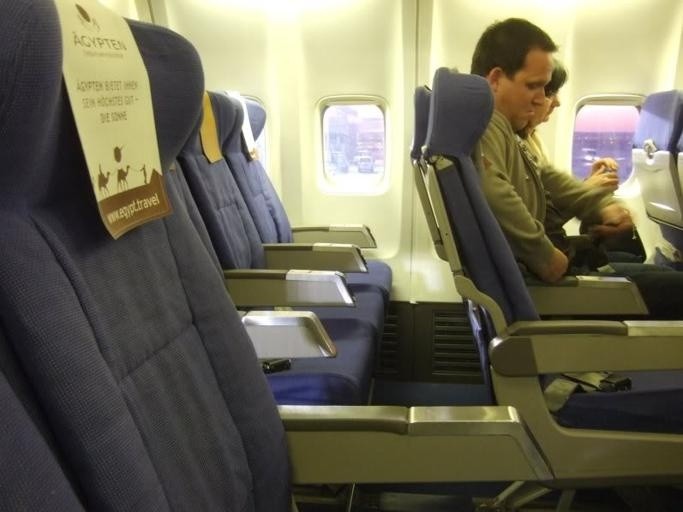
[579,148,600,161]
[357,156,374,172]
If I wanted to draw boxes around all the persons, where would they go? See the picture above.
[519,59,647,264]
[470,18,683,320]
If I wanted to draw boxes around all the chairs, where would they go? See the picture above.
[0,0,683,512]
